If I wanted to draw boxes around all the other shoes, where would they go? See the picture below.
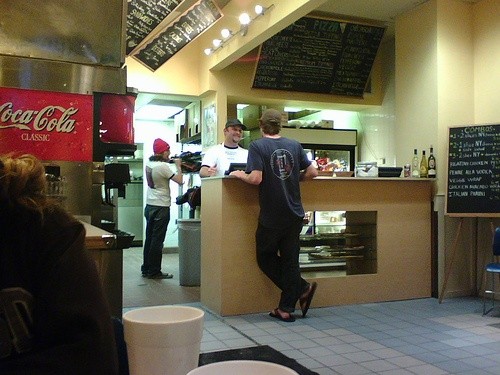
[144,272,172,279]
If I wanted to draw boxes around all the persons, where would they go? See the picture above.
[229,109,317,322]
[0,152,119,375]
[199,120,248,177]
[141,138,182,279]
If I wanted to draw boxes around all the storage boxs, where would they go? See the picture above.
[321,120,334,129]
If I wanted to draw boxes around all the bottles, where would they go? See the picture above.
[404,164,411,177]
[428,147,436,178]
[412,148,420,178]
[420,151,427,178]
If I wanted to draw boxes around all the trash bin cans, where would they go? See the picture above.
[176,218,204,287]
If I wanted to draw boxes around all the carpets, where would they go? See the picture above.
[197,343,319,375]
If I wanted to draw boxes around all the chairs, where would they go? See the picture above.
[483,226,500,315]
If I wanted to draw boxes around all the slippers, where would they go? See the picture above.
[269,308,295,322]
[298,282,316,317]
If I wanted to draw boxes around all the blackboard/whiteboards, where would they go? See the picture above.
[446,121,500,217]
[130,0,225,72]
[251,14,387,100]
[125,0,183,58]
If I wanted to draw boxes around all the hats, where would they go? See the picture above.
[153,138,170,155]
[225,119,247,130]
[261,108,282,125]
